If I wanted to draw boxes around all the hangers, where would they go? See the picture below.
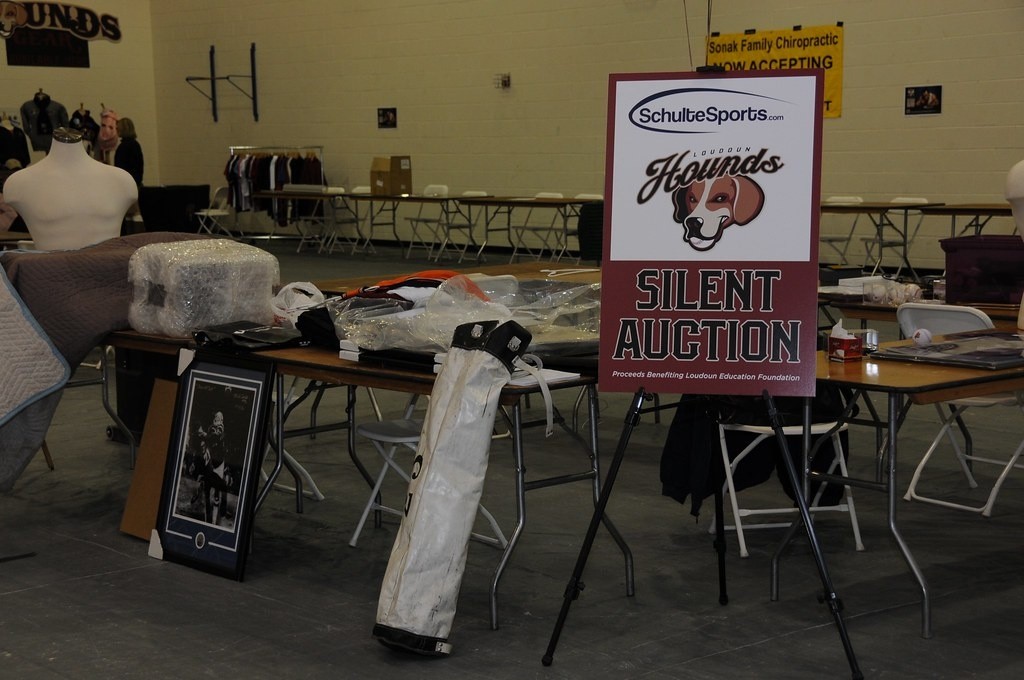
[233,146,318,160]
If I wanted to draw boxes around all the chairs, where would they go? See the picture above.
[17,182,1024,558]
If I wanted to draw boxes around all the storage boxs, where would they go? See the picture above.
[936,232,1023,307]
[369,155,413,197]
[125,239,281,338]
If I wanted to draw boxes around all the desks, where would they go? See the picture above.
[0,191,1024,640]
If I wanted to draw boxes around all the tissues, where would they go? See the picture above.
[828,317,862,364]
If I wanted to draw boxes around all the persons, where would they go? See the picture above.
[114,117,144,188]
[201,411,228,525]
[0,88,119,192]
[2,126,139,251]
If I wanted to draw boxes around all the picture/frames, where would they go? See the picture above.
[145,347,277,583]
